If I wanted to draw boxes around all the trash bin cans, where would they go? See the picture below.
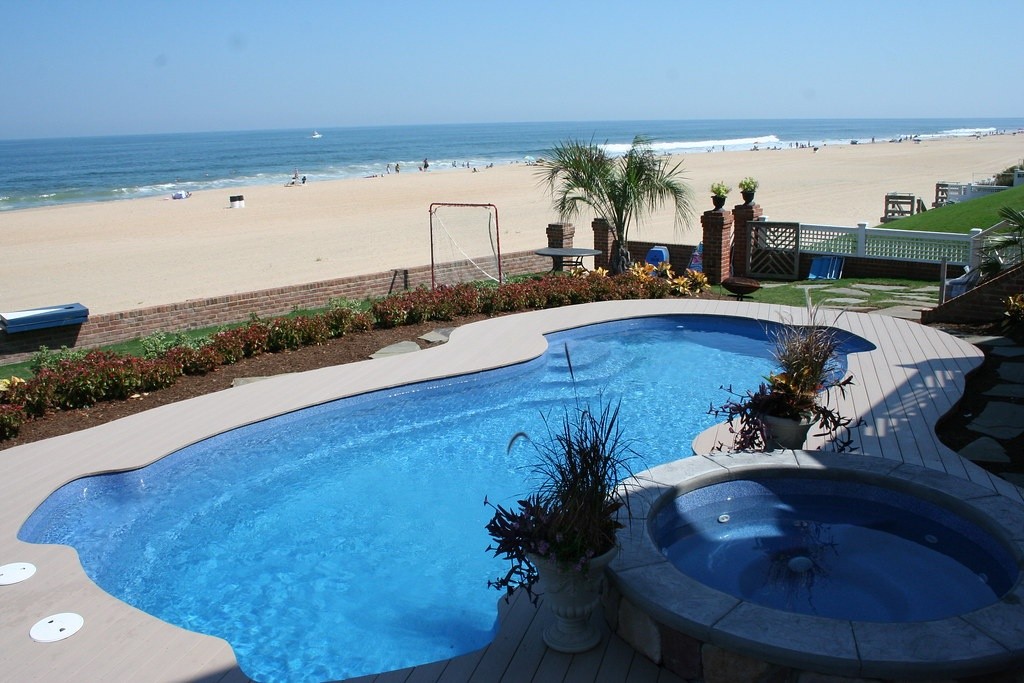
[644,245,670,269]
[229,195,245,208]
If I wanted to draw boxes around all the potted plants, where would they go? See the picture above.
[709,181,733,212]
[706,375,868,454]
[754,286,858,403]
[738,176,760,206]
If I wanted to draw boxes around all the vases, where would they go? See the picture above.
[524,535,622,652]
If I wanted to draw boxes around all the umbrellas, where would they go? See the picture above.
[524,156,534,165]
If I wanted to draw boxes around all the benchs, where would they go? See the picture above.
[879,181,963,222]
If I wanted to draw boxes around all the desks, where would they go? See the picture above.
[535,247,603,275]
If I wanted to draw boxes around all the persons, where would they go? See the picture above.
[890,133,922,144]
[871,136,875,144]
[301,176,306,184]
[750,144,759,151]
[624,149,672,158]
[452,160,493,173]
[533,158,546,166]
[722,145,725,151]
[395,164,400,173]
[708,145,714,152]
[423,158,429,173]
[768,140,810,150]
[294,167,298,178]
[973,128,1005,141]
[291,177,296,184]
[387,164,391,174]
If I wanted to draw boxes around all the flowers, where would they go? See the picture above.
[483,342,675,612]
